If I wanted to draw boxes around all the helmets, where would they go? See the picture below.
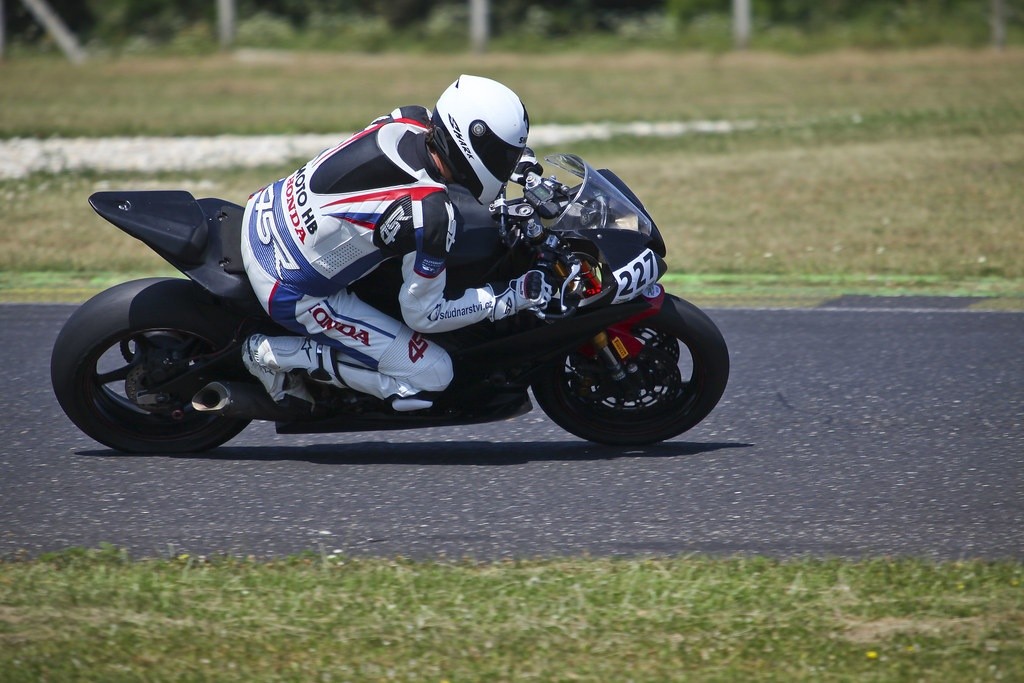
[431,74,528,204]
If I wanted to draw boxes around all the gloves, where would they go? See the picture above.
[509,147,544,187]
[486,270,554,322]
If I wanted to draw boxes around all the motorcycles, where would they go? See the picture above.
[49,153,732,461]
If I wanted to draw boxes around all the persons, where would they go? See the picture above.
[240,74,558,418]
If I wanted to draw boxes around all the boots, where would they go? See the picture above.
[242,334,348,417]
